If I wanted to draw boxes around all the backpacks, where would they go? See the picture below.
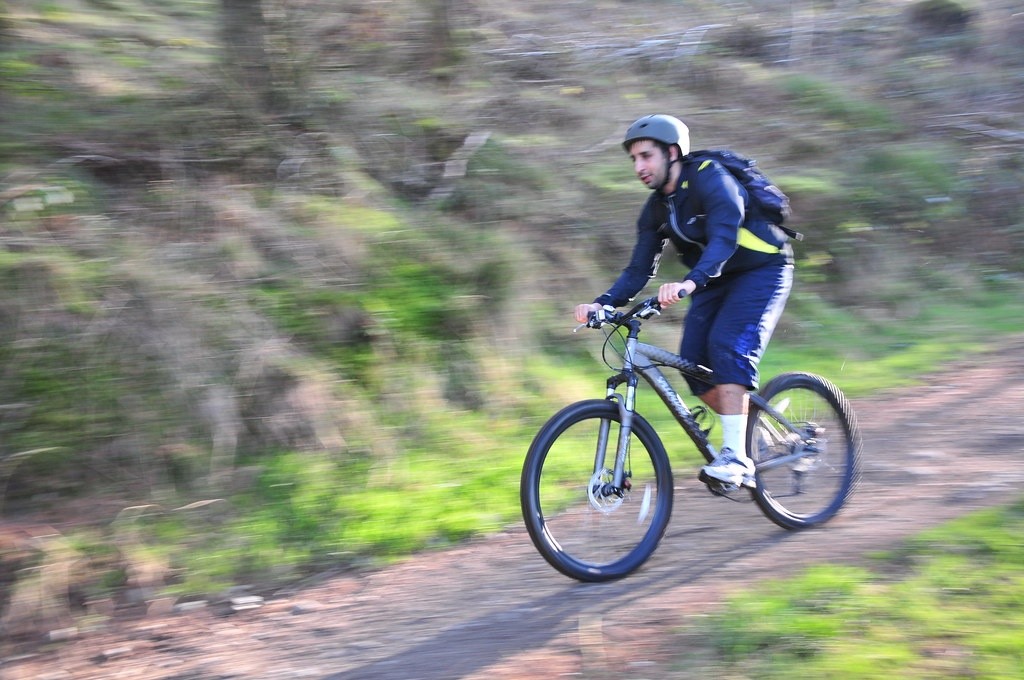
[686,148,795,223]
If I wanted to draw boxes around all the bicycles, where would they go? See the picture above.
[519,286,864,584]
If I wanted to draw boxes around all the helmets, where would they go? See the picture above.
[623,112,692,158]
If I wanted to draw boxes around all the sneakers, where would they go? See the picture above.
[698,447,758,490]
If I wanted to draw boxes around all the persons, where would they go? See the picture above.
[573,114,796,489]
